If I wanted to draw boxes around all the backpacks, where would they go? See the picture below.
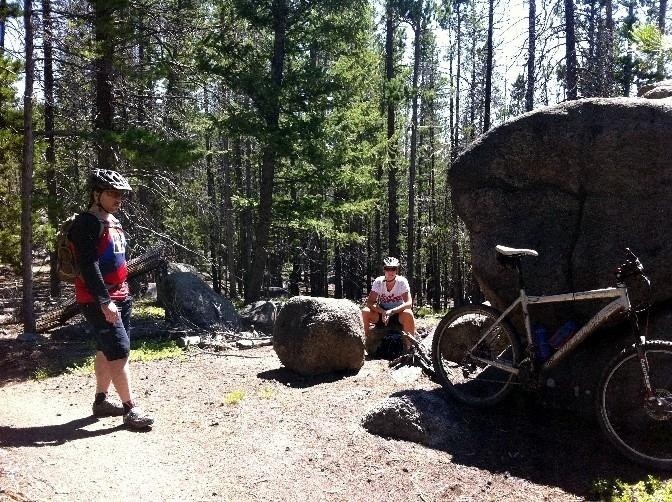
[57,213,105,280]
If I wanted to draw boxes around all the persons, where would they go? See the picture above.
[65,168,153,429]
[359,255,416,357]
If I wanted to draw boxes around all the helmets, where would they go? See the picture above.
[383,257,400,267]
[93,169,132,191]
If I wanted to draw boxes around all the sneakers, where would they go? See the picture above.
[123,412,154,428]
[93,400,123,415]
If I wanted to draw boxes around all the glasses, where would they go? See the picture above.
[384,268,396,271]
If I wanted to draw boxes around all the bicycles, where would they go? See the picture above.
[431,244,672,474]
